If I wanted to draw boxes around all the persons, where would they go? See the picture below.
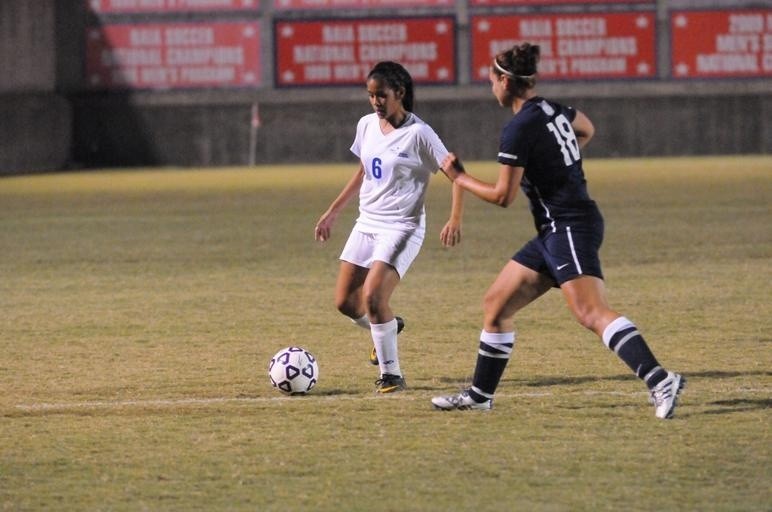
[430,41,685,419]
[315,62,463,394]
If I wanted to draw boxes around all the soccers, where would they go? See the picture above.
[269,347,318,395]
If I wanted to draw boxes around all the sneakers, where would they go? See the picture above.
[370,316,404,365]
[375,373,407,398]
[431,387,492,413]
[651,370,685,419]
